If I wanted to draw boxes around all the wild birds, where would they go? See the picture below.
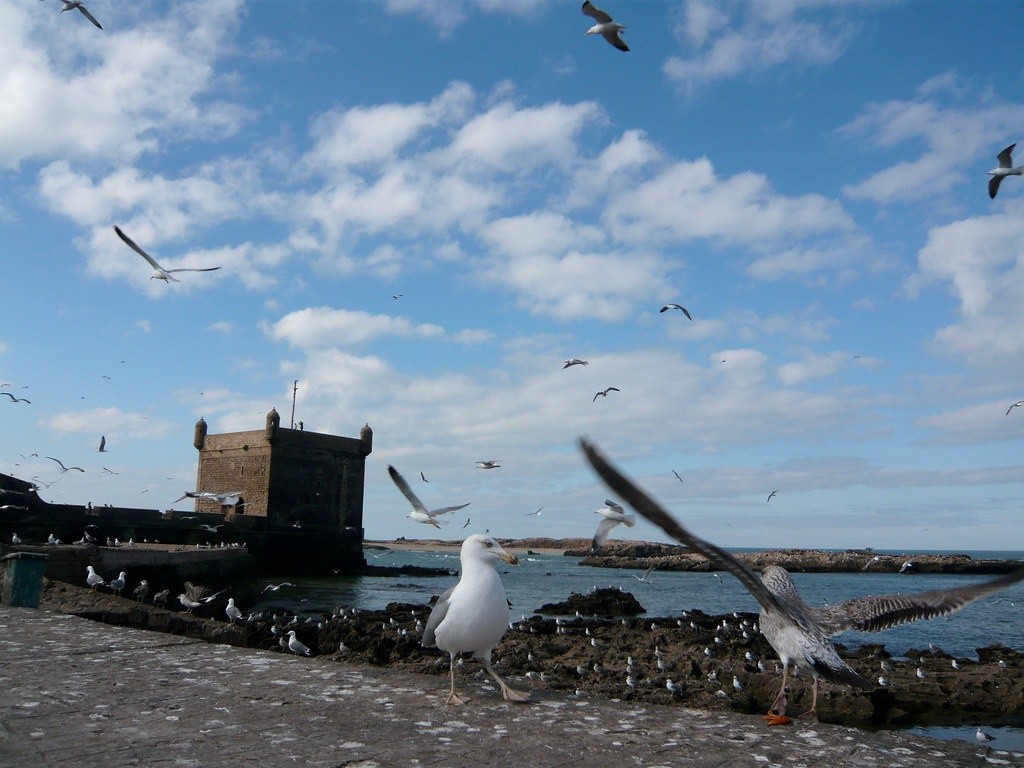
[420,532,532,707]
[561,358,589,370]
[861,554,893,570]
[474,459,504,469]
[85,565,1010,747]
[631,564,657,585]
[766,489,778,502]
[525,505,546,516]
[592,386,621,402]
[112,224,223,283]
[0,362,251,550]
[38,0,106,31]
[580,0,631,52]
[898,557,917,572]
[385,463,474,531]
[1005,400,1024,416]
[574,430,1024,725]
[659,303,693,322]
[986,142,1024,199]
[279,630,312,657]
[671,468,684,484]
[590,498,638,553]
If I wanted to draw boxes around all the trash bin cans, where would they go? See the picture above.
[1,552,50,608]
